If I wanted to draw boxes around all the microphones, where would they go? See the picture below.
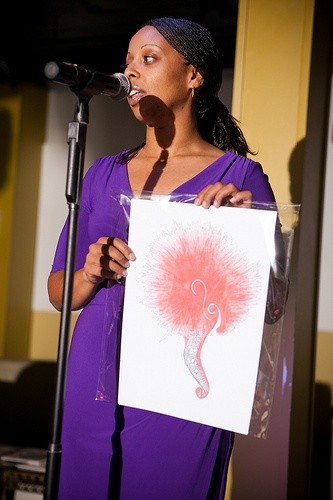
[43,60,133,102]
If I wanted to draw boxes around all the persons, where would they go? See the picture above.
[47,17,290,499]
